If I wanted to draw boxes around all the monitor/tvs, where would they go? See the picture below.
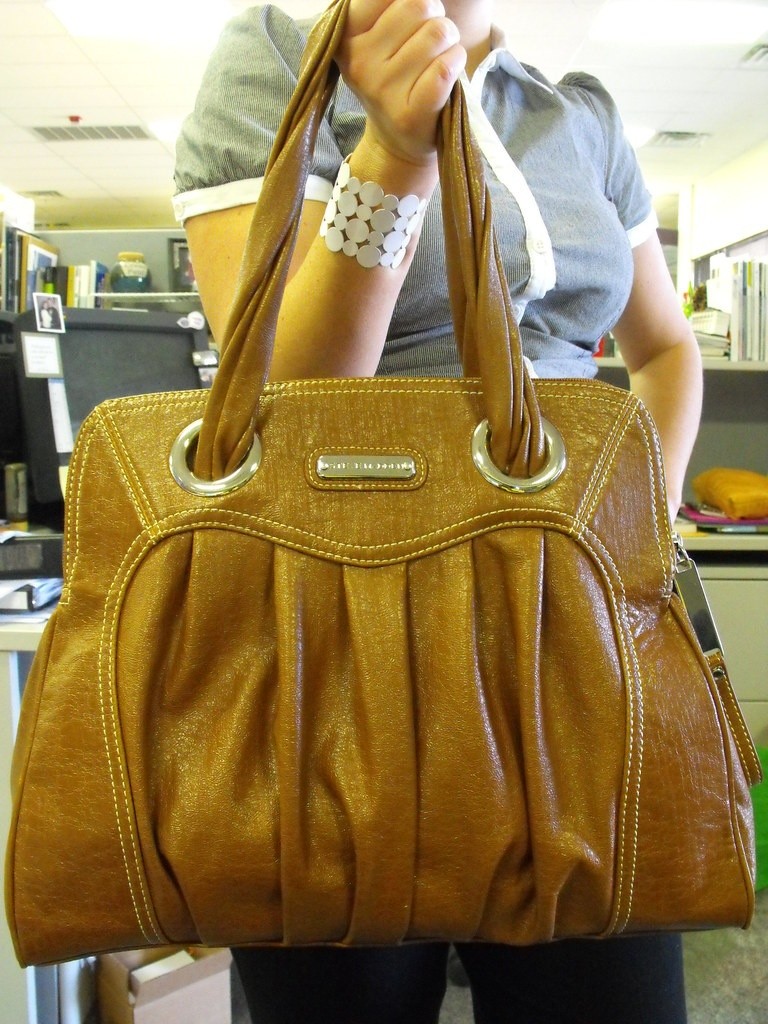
[11,301,222,533]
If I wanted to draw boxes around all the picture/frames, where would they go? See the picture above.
[32,292,66,334]
[168,237,199,292]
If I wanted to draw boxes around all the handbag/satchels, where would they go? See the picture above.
[1,0,755,969]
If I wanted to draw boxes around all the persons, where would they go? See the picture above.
[174,0,704,1024]
[175,246,195,289]
[41,299,61,329]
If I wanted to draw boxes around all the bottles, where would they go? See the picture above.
[110,253,151,292]
[5,463,29,532]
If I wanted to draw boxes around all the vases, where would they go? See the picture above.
[111,252,151,293]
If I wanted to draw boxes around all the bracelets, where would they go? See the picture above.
[320,152,431,269]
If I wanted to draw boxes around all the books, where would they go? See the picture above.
[674,502,768,533]
[0,600,59,624]
[691,254,768,362]
[0,211,109,313]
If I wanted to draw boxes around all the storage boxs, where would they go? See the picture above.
[95,943,233,1024]
